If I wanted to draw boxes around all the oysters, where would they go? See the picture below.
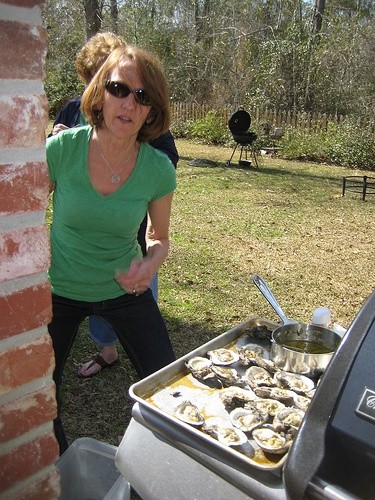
[172,343,319,455]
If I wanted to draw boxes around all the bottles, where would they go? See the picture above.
[312,308,330,328]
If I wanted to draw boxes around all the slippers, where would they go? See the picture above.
[77,348,120,377]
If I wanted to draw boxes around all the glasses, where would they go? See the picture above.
[103,81,154,106]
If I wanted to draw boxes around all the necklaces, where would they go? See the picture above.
[96,141,137,183]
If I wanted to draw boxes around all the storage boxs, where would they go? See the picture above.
[58,437,131,500]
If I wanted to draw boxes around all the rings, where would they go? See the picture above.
[133,286,138,296]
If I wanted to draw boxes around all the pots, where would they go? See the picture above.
[227,107,257,146]
[253,273,342,376]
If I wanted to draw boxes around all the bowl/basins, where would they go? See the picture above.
[238,160,252,168]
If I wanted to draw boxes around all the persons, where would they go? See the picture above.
[47,32,180,380]
[46,47,177,457]
